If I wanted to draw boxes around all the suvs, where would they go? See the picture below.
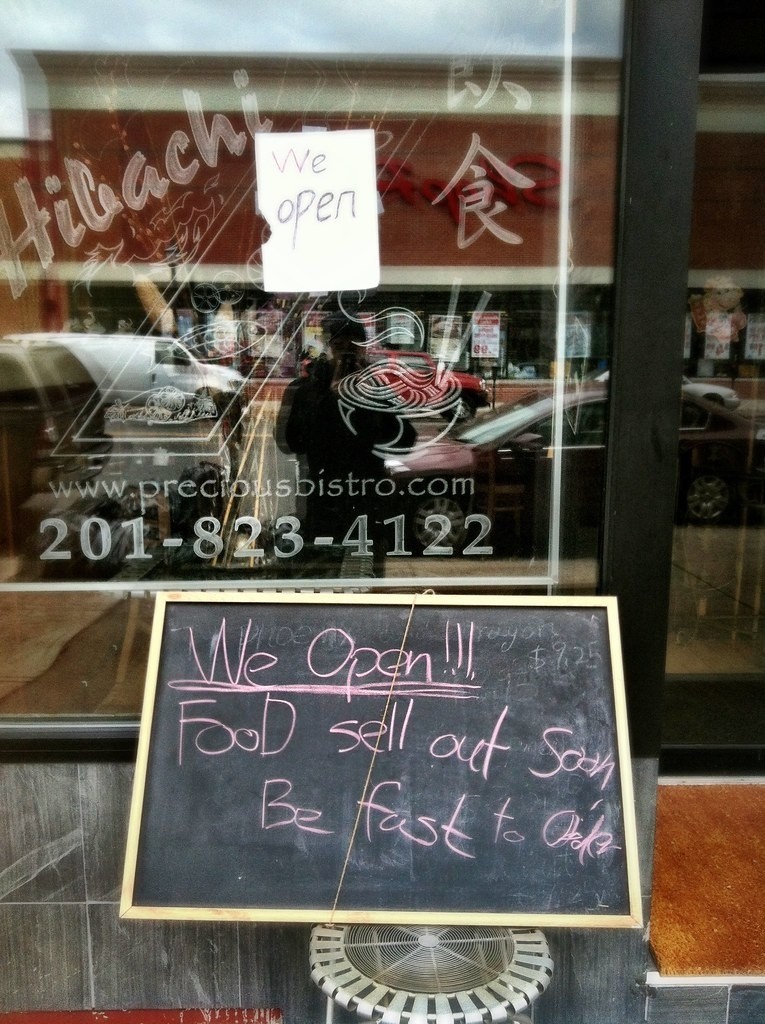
[298,351,492,424]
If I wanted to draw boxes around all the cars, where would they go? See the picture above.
[381,381,764,558]
[594,364,743,411]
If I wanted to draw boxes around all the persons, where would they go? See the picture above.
[272,310,417,578]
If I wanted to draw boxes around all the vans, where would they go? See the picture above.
[6,332,247,415]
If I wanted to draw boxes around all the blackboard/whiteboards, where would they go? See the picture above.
[115,590,645,931]
